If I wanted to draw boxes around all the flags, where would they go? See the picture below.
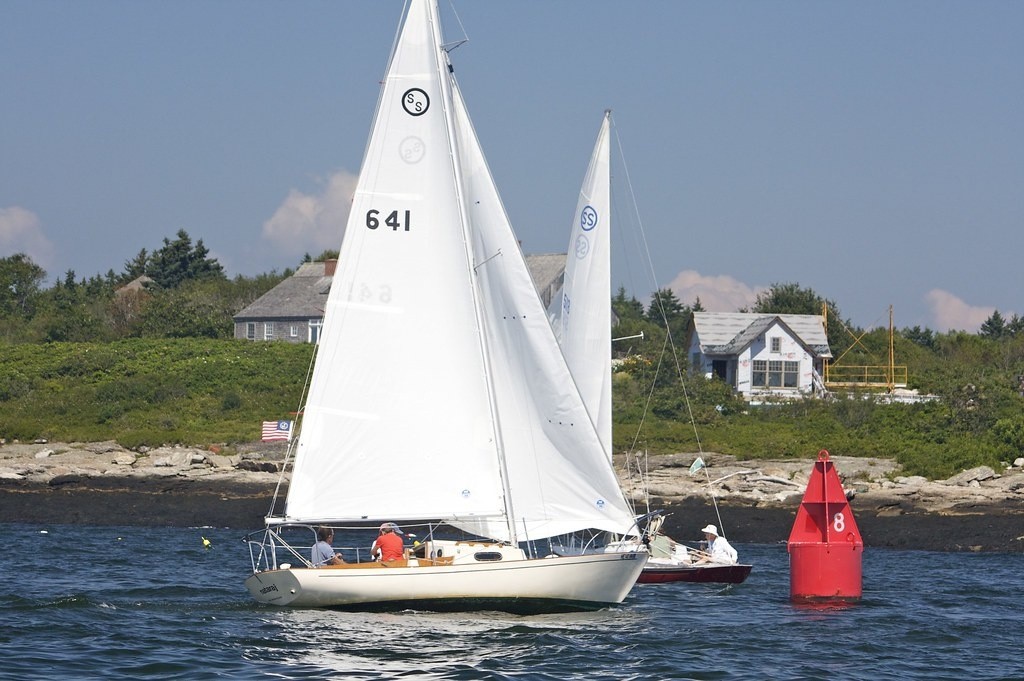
[689,457,704,477]
[260,420,294,442]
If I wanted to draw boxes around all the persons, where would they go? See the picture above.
[695,524,738,565]
[371,523,404,562]
[310,524,347,566]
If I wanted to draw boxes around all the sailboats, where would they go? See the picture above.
[245,1,654,615]
[554,106,753,585]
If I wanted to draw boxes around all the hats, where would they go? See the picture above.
[391,523,403,534]
[379,523,391,531]
[701,525,719,537]
